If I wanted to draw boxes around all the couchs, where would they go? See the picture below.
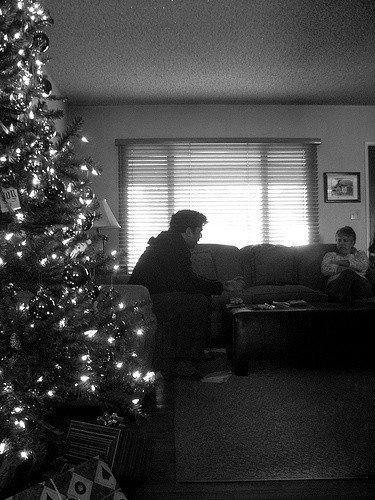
[96,244,375,373]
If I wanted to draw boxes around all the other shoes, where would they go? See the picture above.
[170,366,204,381]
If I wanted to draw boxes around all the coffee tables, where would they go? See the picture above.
[225,303,375,376]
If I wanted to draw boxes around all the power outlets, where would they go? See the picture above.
[350,209,358,219]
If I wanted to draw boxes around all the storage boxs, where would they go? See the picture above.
[0,455,130,500]
[64,420,159,480]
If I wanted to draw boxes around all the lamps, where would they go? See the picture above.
[92,199,122,253]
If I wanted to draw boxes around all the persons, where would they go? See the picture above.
[127,210,245,381]
[319,227,375,302]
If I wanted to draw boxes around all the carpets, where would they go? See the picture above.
[174,361,375,483]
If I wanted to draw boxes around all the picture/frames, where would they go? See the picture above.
[323,172,361,203]
